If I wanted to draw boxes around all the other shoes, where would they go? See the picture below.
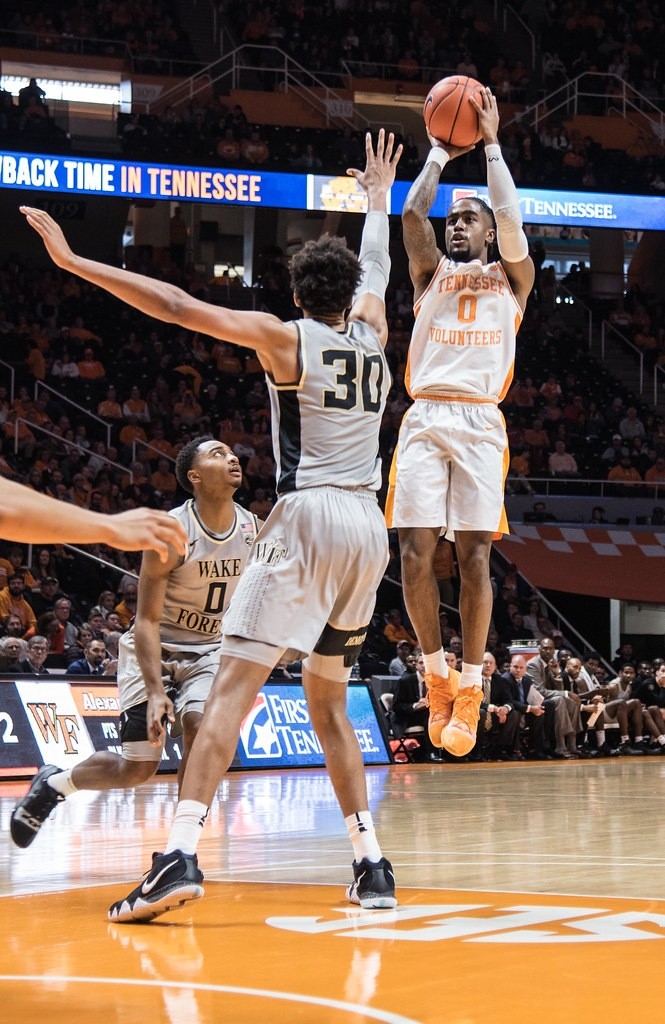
[478,736,665,760]
[424,753,443,762]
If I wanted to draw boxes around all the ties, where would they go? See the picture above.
[485,679,492,731]
[517,681,526,729]
[422,681,426,699]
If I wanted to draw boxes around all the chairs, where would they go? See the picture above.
[381,692,429,763]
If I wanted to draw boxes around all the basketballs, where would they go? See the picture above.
[423,74,486,147]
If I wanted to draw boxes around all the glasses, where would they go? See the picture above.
[407,660,417,663]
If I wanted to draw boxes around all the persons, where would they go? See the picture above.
[0,0,665,923]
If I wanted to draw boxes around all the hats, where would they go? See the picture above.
[613,434,621,441]
[553,631,563,638]
[397,639,409,648]
[575,396,582,400]
[42,576,59,583]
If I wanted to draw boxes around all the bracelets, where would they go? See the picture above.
[425,146,450,172]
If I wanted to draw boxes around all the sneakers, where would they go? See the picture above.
[440,684,484,756]
[346,857,398,908]
[106,849,204,922]
[9,765,66,848]
[413,666,460,747]
